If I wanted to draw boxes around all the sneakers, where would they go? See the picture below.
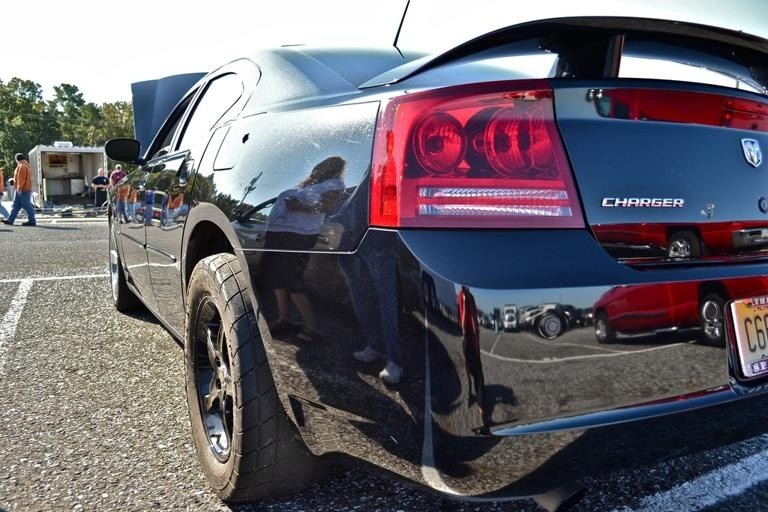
[297,327,323,342]
[379,359,405,385]
[269,319,289,331]
[352,346,392,363]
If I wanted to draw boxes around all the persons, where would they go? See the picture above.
[1,153,37,226]
[91,164,127,215]
[254,156,346,344]
[455,286,486,417]
[112,179,185,229]
[4,176,15,201]
[0,170,9,219]
[325,163,410,388]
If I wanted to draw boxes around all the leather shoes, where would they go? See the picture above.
[23,222,35,227]
[1,219,12,225]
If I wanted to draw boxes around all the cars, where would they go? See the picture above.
[592,276,768,346]
[592,223,768,261]
[419,263,458,315]
[230,184,424,324]
[110,16,768,503]
[481,303,593,340]
[135,189,168,225]
